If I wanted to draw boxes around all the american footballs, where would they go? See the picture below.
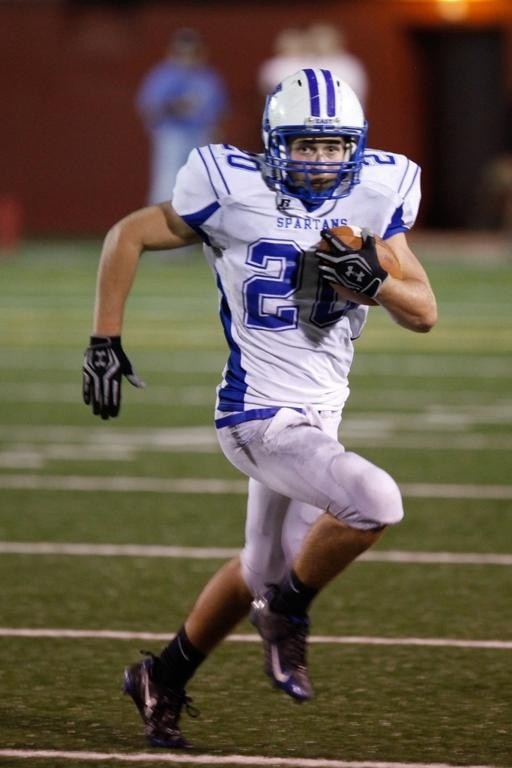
[320,225,400,306]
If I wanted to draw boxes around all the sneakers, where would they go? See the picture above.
[248,588,315,703]
[122,658,196,750]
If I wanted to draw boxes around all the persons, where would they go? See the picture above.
[133,28,227,224]
[83,66,439,749]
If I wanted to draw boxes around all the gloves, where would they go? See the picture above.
[82,335,146,421]
[311,225,390,301]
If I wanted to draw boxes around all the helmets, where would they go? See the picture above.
[260,67,371,203]
